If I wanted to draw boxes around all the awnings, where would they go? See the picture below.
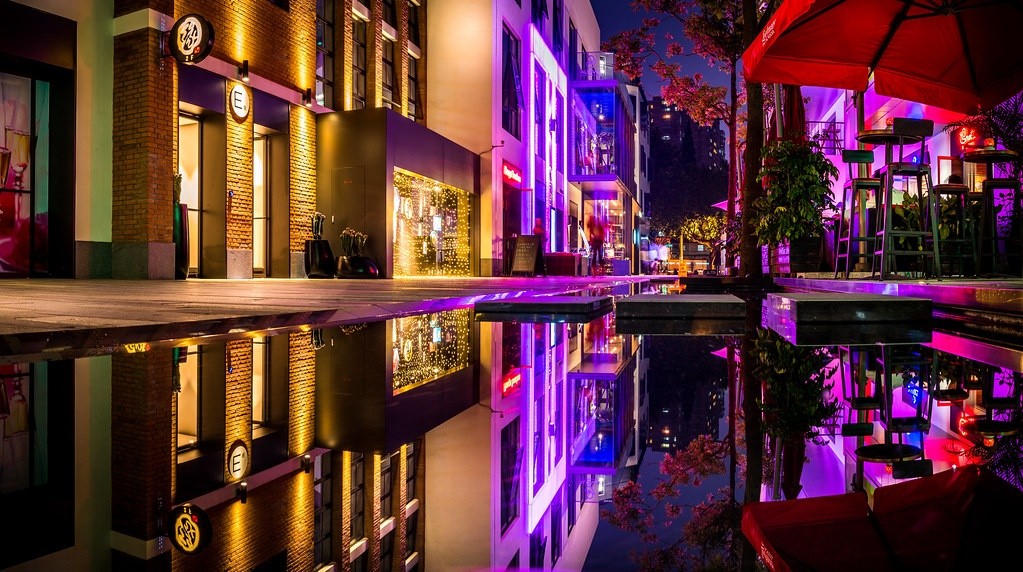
[742,0,1023,119]
[741,463,1023,572]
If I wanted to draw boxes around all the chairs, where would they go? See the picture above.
[832,148,892,279]
[874,117,945,279]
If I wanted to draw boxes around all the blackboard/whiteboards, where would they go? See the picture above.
[510,234,546,278]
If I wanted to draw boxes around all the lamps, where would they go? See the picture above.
[301,455,311,473]
[302,88,311,108]
[235,480,248,504]
[238,59,250,82]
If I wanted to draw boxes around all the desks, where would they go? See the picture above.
[957,149,1018,277]
[834,343,1022,478]
[854,129,923,276]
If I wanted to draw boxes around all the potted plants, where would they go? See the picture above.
[302,211,335,278]
[336,226,378,280]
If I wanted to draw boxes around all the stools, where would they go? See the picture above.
[930,182,968,274]
